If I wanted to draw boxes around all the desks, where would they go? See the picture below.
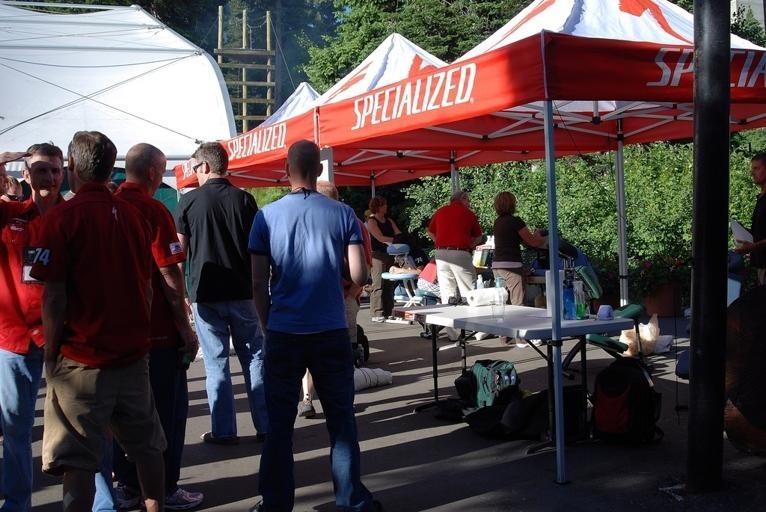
[389,301,637,457]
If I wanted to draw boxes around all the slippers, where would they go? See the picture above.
[203,431,242,445]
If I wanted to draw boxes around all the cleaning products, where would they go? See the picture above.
[490,276,506,324]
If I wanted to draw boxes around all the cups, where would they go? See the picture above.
[597,303,615,320]
[490,295,505,325]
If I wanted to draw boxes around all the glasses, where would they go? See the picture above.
[192,162,205,174]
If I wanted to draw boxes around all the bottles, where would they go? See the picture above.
[179,337,200,370]
[356,343,366,368]
[343,284,359,353]
[544,269,592,321]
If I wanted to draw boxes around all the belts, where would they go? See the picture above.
[436,247,467,251]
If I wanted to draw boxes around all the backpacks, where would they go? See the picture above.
[470,359,518,409]
[588,356,664,445]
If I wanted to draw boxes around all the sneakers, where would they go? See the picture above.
[112,484,145,512]
[163,487,204,511]
[297,402,317,418]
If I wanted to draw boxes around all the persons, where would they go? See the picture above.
[176,141,269,445]
[0,164,9,202]
[1,144,69,511]
[30,130,168,512]
[296,184,373,418]
[734,153,765,285]
[0,176,20,202]
[360,190,558,323]
[248,139,384,511]
[20,168,30,200]
[115,142,206,512]
